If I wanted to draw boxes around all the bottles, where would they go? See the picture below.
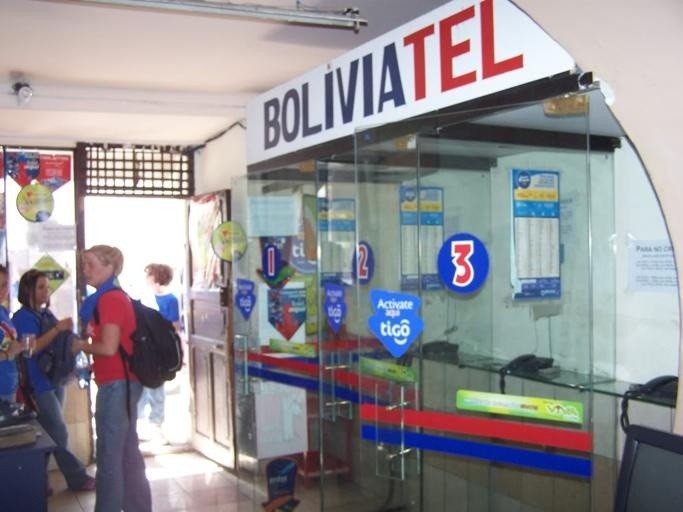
[72,347,91,387]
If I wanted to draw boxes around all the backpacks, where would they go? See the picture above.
[24,306,75,385]
[94,287,182,388]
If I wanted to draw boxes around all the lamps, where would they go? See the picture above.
[61,0,368,34]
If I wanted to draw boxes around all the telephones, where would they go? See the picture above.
[509,354,554,377]
[644,375,677,397]
[423,341,459,355]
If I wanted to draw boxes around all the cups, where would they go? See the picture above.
[21,334,35,358]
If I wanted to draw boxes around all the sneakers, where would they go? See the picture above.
[45,482,53,496]
[81,477,95,489]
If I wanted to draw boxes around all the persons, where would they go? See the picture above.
[11,269,95,495]
[68,242,153,511]
[132,262,180,448]
[0,263,36,413]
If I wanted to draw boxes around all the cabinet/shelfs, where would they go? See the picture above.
[0,400,58,512]
